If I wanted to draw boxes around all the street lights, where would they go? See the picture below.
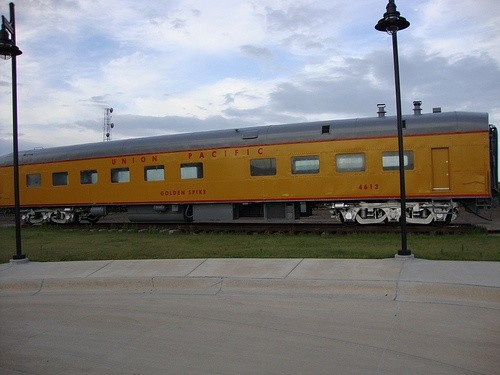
[375,0,412,255]
[0,2,26,259]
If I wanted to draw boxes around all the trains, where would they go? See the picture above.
[1,100,499,230]
